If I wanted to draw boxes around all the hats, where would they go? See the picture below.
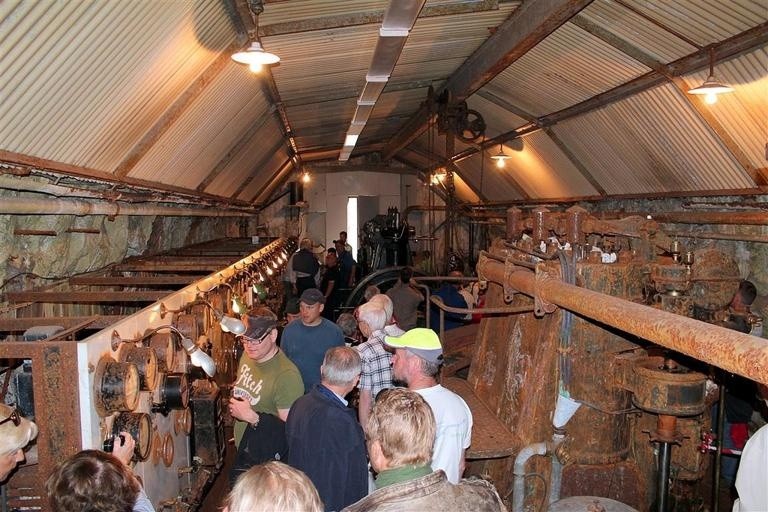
[384,327,445,365]
[296,288,327,305]
[332,240,345,245]
[236,308,278,339]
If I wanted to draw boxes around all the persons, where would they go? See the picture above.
[723,277,764,512]
[341,266,486,336]
[0,402,39,483]
[284,345,369,512]
[280,288,345,395]
[287,231,356,306]
[216,461,325,512]
[384,328,474,485]
[339,387,509,512]
[44,431,157,512]
[354,302,408,440]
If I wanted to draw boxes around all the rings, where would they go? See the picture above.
[227,307,305,453]
[230,409,232,413]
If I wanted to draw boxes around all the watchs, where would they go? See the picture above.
[251,416,259,430]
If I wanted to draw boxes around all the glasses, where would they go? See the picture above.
[1,409,23,428]
[241,328,271,345]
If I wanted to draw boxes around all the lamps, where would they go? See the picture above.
[241,235,299,299]
[685,45,735,105]
[490,144,513,168]
[195,291,246,334]
[160,300,247,337]
[230,1,280,74]
[110,325,216,378]
[215,280,247,315]
